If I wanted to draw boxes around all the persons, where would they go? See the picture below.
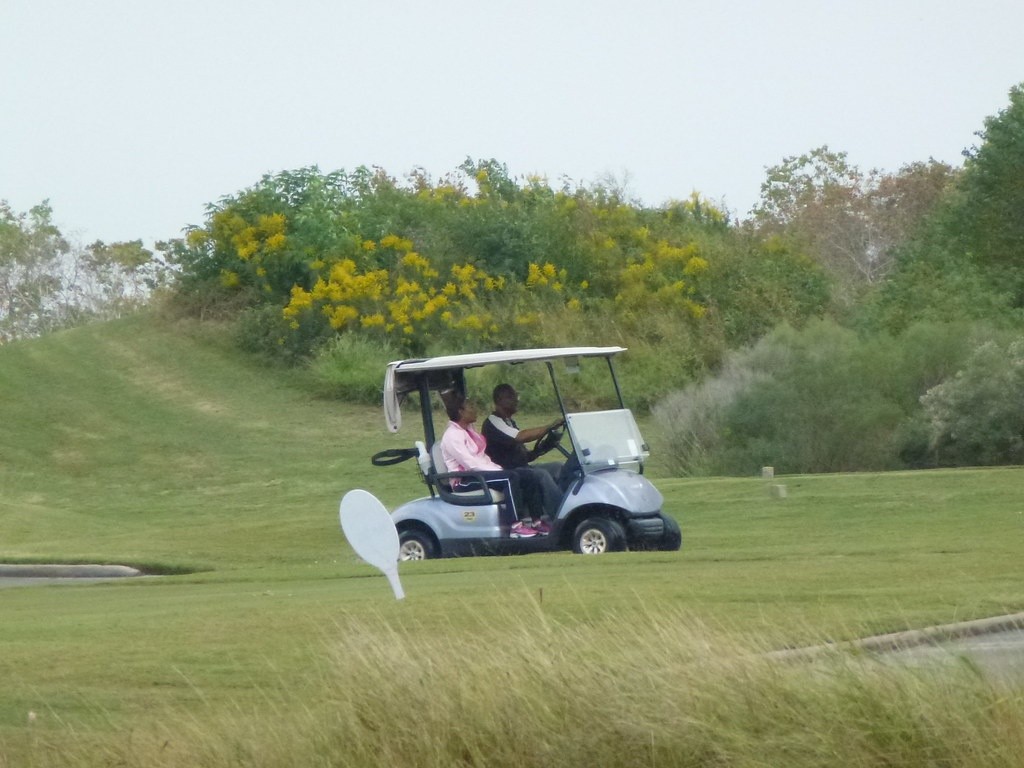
[481,384,576,518]
[441,392,552,538]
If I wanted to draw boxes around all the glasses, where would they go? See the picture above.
[500,395,520,401]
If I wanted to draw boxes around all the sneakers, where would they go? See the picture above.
[531,520,552,536]
[509,521,538,539]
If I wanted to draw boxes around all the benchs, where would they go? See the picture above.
[430,441,506,505]
